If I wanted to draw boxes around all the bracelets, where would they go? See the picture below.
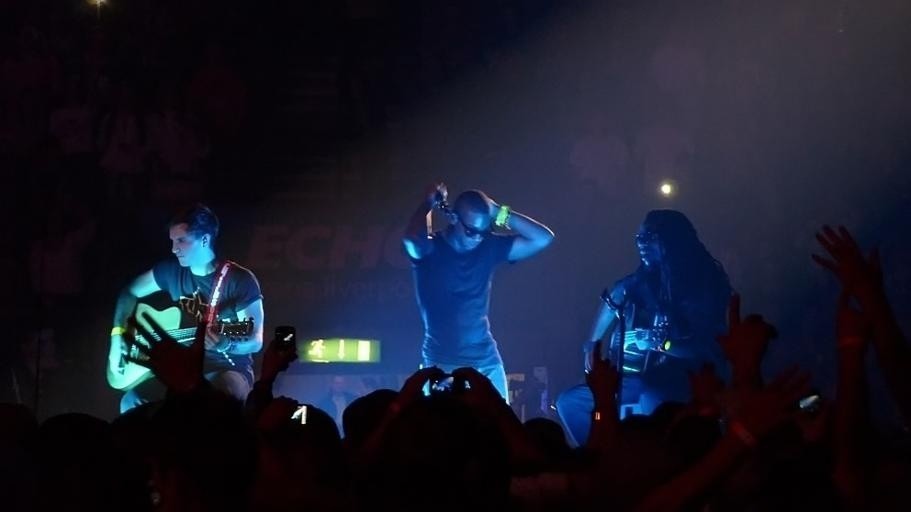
[495,204,512,226]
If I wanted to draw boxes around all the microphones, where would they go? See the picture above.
[433,189,458,227]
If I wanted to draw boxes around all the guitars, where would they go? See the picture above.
[106,301,254,393]
[606,298,695,376]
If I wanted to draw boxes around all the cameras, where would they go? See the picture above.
[428,372,472,397]
[290,404,309,427]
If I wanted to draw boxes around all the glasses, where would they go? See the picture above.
[459,219,493,239]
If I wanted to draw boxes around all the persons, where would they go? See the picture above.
[402,181,557,411]
[1,219,909,509]
[109,202,266,412]
[554,208,736,449]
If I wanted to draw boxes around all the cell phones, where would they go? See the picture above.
[798,391,820,419]
[275,325,297,348]
